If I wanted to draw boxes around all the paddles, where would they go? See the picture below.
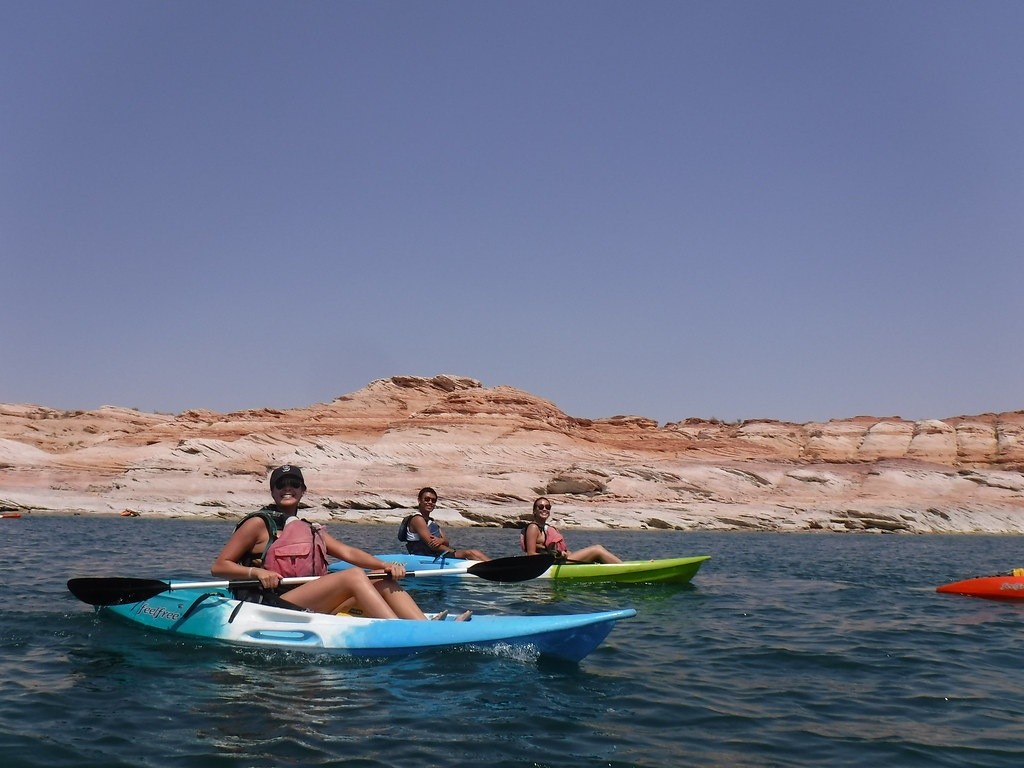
[547,542,596,565]
[66,553,555,606]
[432,550,450,564]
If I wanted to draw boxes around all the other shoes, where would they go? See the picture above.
[432,610,449,621]
[451,610,473,621]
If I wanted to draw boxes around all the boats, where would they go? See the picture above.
[936,568,1024,600]
[95,580,636,662]
[328,553,711,588]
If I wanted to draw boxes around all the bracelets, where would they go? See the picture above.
[248,567,256,579]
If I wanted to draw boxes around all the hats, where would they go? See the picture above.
[270,464,304,490]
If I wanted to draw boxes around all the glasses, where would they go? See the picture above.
[275,480,301,490]
[424,497,437,504]
[536,504,552,510]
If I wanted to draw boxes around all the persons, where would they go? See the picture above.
[406,487,492,561]
[526,497,623,564]
[210,464,472,622]
